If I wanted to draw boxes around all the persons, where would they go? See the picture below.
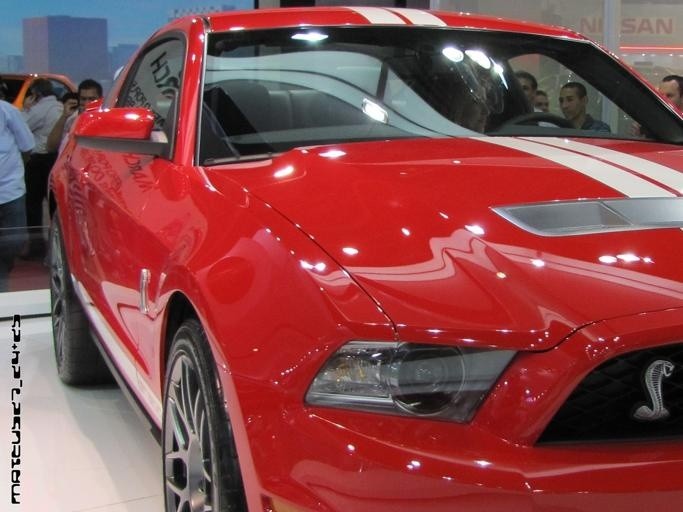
[61,93,80,113]
[1,76,38,278]
[47,78,105,162]
[535,89,551,115]
[432,69,493,136]
[18,77,65,264]
[514,70,559,131]
[631,76,683,149]
[560,81,613,135]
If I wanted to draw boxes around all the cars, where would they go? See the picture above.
[48,6,683,512]
[0,73,77,112]
